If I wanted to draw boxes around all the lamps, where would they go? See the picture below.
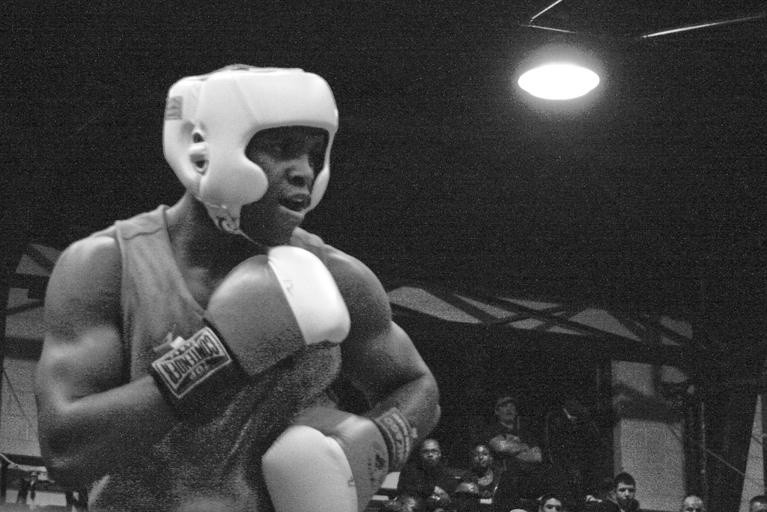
[511,40,600,102]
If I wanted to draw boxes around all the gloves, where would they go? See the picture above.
[257,395,417,512]
[146,241,353,413]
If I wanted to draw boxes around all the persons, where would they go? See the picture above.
[33,63,443,512]
[680,492,704,512]
[375,387,644,511]
[748,496,766,511]
